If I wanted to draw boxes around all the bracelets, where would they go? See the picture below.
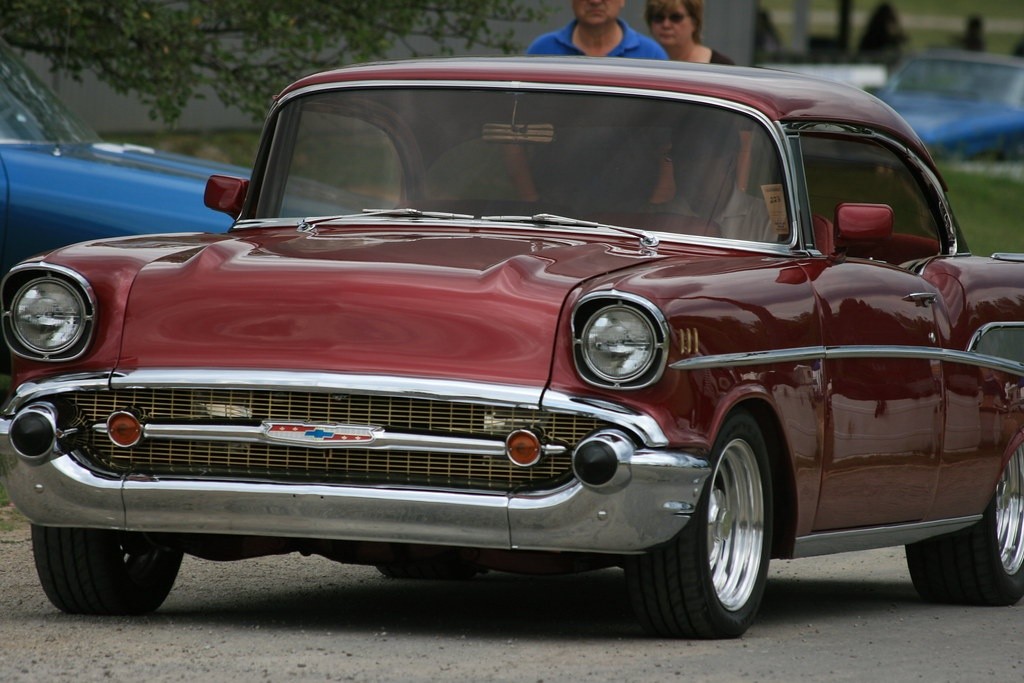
[737,186,746,192]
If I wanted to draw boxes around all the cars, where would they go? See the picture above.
[0,36,401,377]
[3,55,1024,642]
[814,48,1024,168]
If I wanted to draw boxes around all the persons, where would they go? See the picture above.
[644,0,757,214]
[496,0,672,211]
[648,109,778,246]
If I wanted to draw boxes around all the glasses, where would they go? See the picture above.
[651,12,691,23]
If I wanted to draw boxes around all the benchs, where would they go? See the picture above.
[863,231,942,264]
